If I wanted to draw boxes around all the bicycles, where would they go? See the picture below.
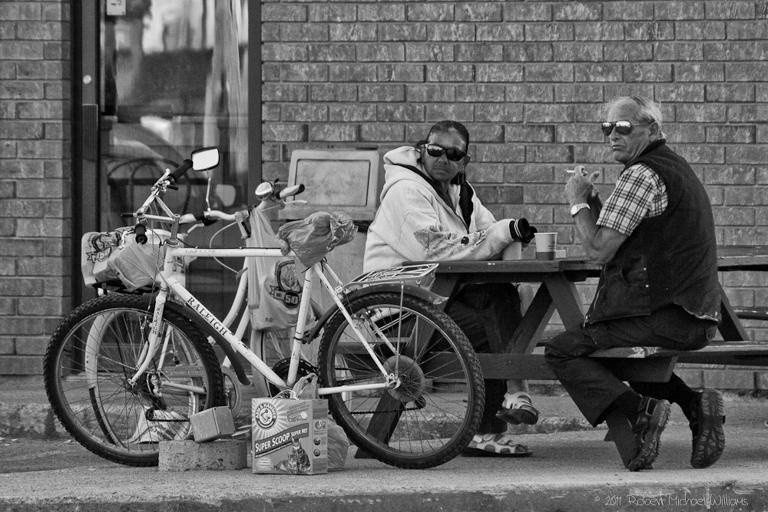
[41,158,486,469]
[82,146,336,454]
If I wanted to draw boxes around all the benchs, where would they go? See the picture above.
[603,337,766,471]
[317,337,426,461]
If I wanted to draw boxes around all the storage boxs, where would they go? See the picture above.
[249,395,328,477]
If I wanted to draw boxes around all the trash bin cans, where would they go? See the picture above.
[278,148,386,377]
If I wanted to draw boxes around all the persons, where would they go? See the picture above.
[542,92,727,472]
[360,119,540,458]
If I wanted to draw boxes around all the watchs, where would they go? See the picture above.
[568,202,591,218]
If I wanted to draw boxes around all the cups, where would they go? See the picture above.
[533,231,558,260]
[502,240,522,261]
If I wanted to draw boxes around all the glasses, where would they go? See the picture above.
[420,142,465,161]
[601,122,656,135]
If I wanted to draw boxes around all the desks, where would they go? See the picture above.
[354,254,767,469]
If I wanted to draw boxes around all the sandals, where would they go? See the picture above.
[688,390,726,468]
[624,398,671,472]
[495,391,539,426]
[462,433,534,457]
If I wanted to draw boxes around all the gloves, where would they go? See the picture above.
[509,217,536,245]
